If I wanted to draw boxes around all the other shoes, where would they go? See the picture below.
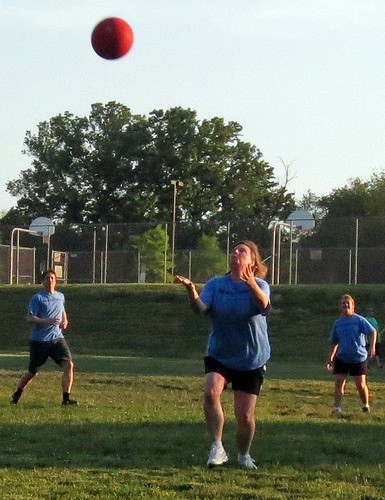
[10,391,21,405]
[61,399,79,406]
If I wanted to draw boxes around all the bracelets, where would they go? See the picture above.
[327,360,334,365]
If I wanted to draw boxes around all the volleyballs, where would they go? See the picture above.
[91,15,134,61]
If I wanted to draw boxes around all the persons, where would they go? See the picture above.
[326,294,377,413]
[176,240,271,470]
[10,270,77,406]
[355,306,385,368]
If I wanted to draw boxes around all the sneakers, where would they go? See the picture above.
[237,453,258,470]
[206,444,229,466]
